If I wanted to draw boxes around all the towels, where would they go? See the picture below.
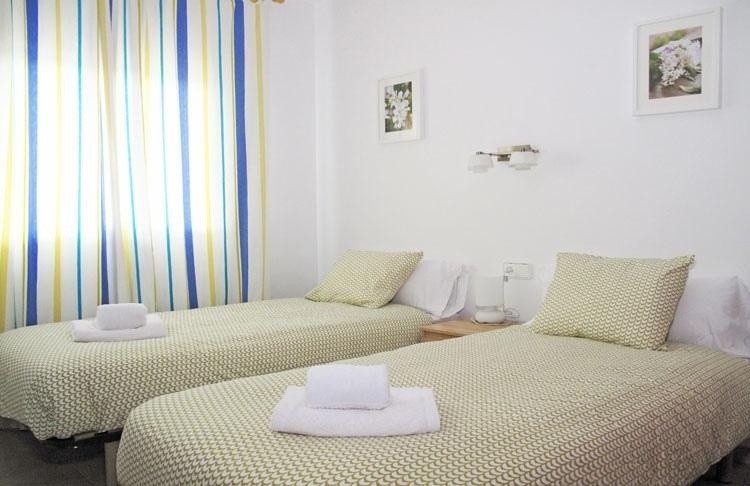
[269,384,441,438]
[68,311,170,342]
[304,364,390,410]
[95,303,148,330]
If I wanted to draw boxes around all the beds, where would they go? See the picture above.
[1,296,460,486]
[115,324,750,485]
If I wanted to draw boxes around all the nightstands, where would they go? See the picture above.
[421,319,523,342]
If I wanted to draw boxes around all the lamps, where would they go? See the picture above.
[467,144,539,174]
[475,278,505,323]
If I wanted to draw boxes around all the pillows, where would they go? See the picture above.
[525,274,750,359]
[304,247,423,310]
[527,252,695,352]
[392,258,481,323]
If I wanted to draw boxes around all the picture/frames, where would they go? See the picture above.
[632,5,723,116]
[378,69,425,145]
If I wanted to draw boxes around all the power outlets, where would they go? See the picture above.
[504,262,533,280]
